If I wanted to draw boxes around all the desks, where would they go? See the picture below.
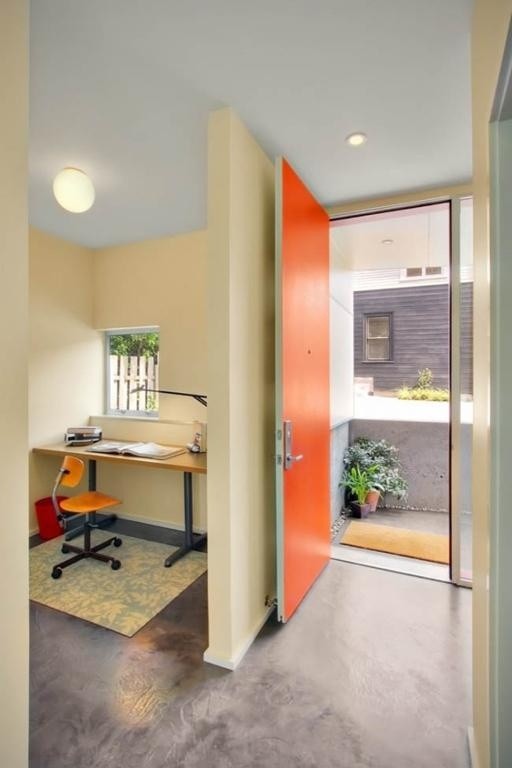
[34,438,209,567]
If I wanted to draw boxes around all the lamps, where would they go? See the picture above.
[131,384,209,416]
[53,166,97,216]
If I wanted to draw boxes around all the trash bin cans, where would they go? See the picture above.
[34,495,69,539]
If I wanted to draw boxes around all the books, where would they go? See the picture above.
[85,438,187,461]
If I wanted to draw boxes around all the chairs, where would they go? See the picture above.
[50,454,122,578]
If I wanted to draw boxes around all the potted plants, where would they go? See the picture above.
[343,439,405,517]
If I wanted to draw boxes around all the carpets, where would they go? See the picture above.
[337,518,450,565]
[29,525,211,638]
